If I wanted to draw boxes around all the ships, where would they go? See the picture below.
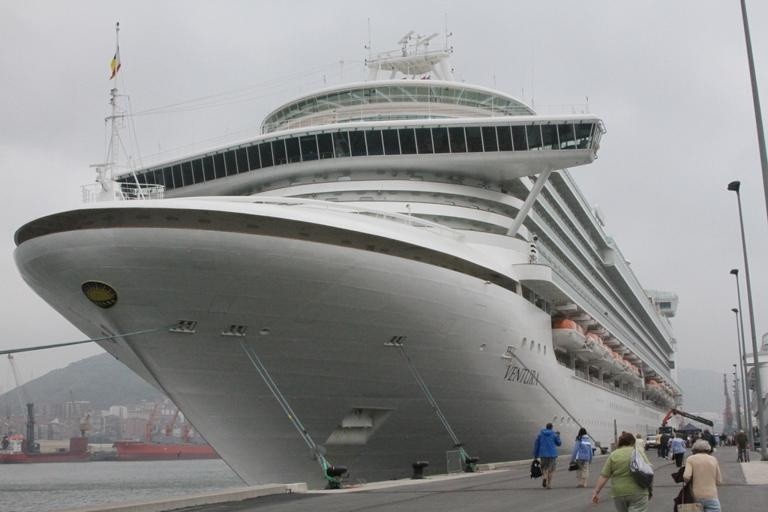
[12,9,714,488]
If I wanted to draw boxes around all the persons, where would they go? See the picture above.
[652,426,751,468]
[682,438,722,512]
[569,427,594,488]
[590,432,654,512]
[634,433,645,452]
[533,422,562,489]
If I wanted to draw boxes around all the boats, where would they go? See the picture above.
[0,400,222,463]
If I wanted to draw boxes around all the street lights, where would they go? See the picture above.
[727,180,767,462]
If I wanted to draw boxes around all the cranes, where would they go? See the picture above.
[655,401,714,448]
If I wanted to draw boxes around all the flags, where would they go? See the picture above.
[108,47,122,79]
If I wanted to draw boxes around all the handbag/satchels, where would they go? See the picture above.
[569,460,578,471]
[677,502,704,512]
[630,448,654,488]
[530,460,541,477]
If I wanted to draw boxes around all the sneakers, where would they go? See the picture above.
[543,477,547,487]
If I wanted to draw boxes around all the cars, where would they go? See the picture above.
[645,435,656,451]
